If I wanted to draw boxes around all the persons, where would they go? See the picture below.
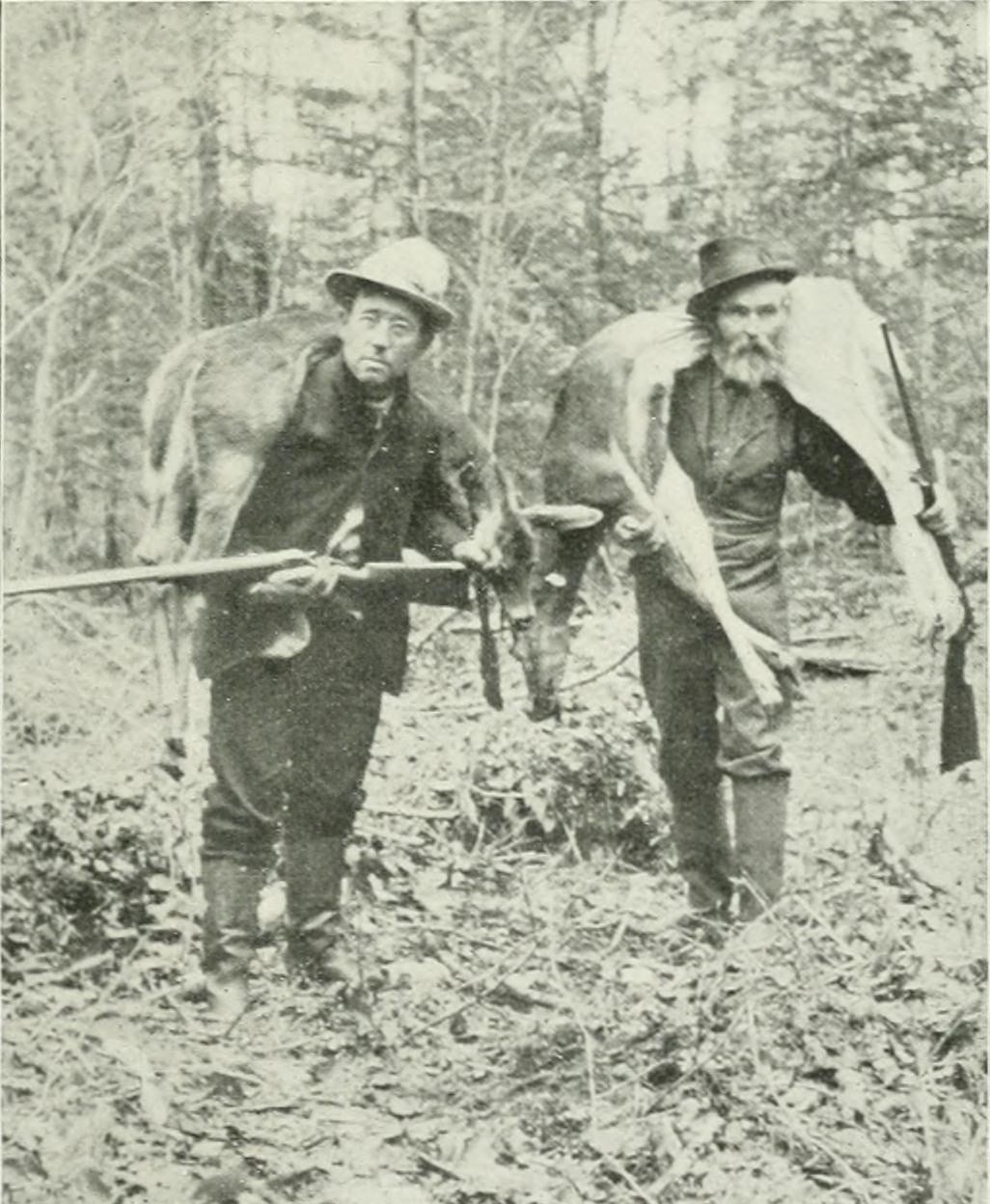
[192,239,533,1031]
[613,239,957,919]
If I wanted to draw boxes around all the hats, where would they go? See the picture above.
[685,237,796,323]
[323,235,455,327]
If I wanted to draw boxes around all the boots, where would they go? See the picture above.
[674,804,729,923]
[284,834,360,985]
[200,854,264,1011]
[732,772,784,920]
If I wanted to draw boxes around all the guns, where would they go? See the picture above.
[879,316,982,775]
[5,548,472,618]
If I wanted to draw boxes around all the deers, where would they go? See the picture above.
[134,306,606,890]
[505,276,969,720]
